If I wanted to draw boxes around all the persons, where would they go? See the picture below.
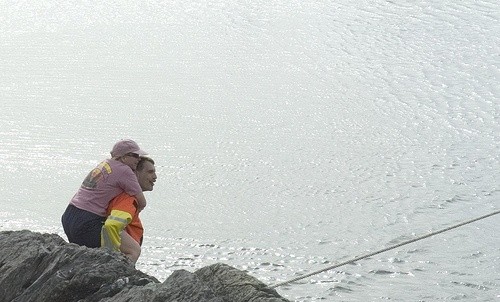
[61,138,150,250]
[101,155,158,265]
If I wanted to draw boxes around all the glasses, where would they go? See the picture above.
[128,153,139,158]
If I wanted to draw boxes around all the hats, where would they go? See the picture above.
[110,139,149,160]
